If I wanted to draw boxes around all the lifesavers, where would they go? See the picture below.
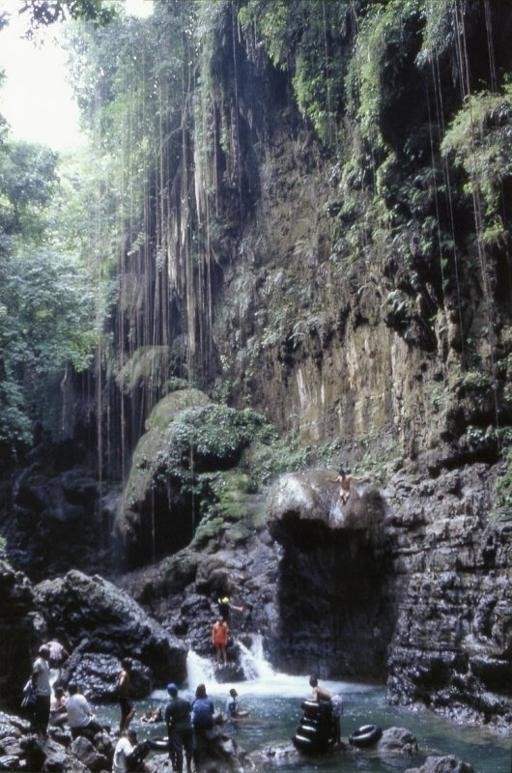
[349,724,381,745]
[148,735,170,749]
[291,701,332,756]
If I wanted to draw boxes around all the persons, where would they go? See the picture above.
[218,597,242,624]
[307,676,343,745]
[327,470,364,506]
[31,638,239,773]
[212,616,228,663]
